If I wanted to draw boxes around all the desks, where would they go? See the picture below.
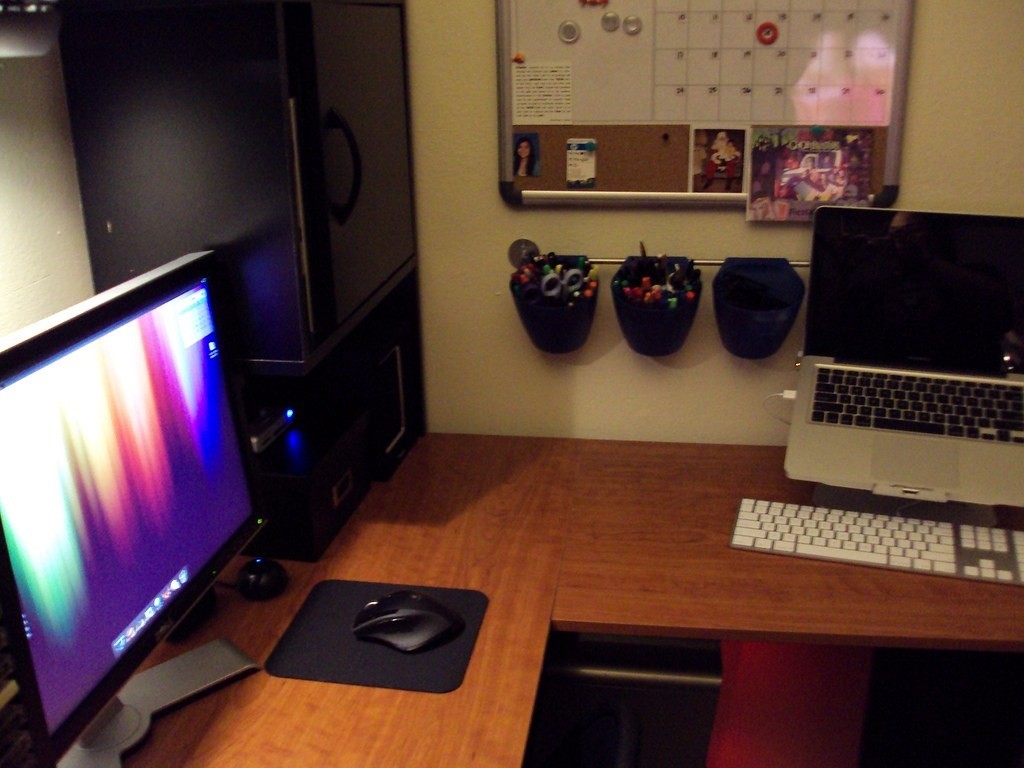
[0,432,1024,768]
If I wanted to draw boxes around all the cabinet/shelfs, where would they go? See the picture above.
[54,0,427,561]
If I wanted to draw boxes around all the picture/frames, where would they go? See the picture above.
[494,0,916,213]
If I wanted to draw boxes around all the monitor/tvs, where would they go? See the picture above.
[0,250,263,768]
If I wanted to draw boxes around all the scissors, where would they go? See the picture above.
[540,268,583,296]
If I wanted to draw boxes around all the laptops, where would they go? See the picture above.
[787,206,1024,507]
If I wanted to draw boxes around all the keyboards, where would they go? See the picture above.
[729,498,1024,588]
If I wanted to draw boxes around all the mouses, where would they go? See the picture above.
[351,589,467,656]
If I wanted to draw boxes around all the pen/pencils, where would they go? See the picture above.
[509,241,608,302]
[612,239,702,309]
